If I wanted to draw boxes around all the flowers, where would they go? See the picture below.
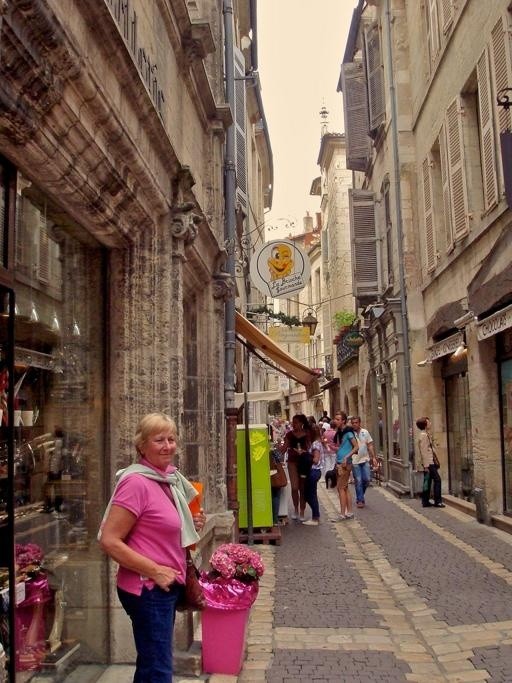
[12,543,43,570]
[208,543,265,580]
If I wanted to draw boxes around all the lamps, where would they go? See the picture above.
[454,311,474,330]
[302,307,318,335]
[455,341,465,358]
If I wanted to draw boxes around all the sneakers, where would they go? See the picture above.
[355,499,365,509]
[422,500,447,509]
[331,512,354,523]
[291,512,320,526]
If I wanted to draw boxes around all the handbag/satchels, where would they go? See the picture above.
[433,452,441,470]
[175,546,208,614]
[269,450,287,489]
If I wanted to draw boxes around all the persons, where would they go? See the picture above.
[415,417,446,507]
[268,412,377,526]
[100,411,206,683]
[40,425,65,514]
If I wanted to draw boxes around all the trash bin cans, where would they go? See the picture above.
[198,570,259,675]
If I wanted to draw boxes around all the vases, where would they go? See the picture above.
[334,326,351,344]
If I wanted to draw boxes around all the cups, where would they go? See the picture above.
[0,409,35,427]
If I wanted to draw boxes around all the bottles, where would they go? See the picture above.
[5,290,84,339]
